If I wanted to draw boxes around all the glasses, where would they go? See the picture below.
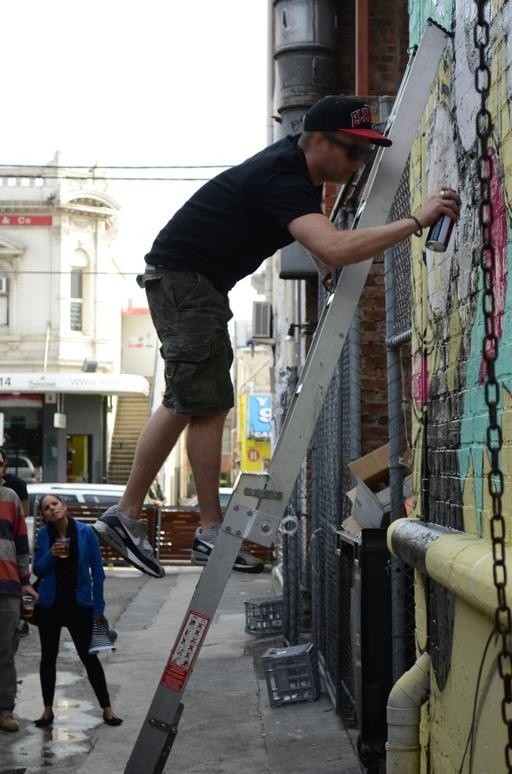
[326,133,376,162]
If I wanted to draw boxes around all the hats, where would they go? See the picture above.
[88,617,118,654]
[304,94,393,147]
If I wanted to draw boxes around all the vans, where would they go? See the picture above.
[6,454,38,486]
[26,484,159,551]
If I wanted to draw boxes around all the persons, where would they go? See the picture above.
[3,453,29,517]
[22,494,123,726]
[93,93,462,578]
[0,450,40,732]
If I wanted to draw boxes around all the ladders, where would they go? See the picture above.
[123,17,452,774]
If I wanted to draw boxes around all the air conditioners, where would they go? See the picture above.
[252,301,274,344]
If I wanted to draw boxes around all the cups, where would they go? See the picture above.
[21,594,36,619]
[56,534,70,560]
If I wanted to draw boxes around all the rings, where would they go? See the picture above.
[440,190,445,199]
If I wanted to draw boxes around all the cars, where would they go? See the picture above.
[187,489,234,507]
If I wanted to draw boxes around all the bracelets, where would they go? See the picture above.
[407,215,422,237]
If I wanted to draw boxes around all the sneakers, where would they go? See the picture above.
[1,716,19,731]
[36,712,54,726]
[91,505,165,579]
[191,526,264,572]
[102,711,123,726]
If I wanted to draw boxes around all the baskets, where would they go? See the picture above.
[261,642,322,708]
[243,595,284,635]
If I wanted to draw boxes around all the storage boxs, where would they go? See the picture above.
[341,442,421,540]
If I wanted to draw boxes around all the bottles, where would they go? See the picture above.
[425,184,463,252]
